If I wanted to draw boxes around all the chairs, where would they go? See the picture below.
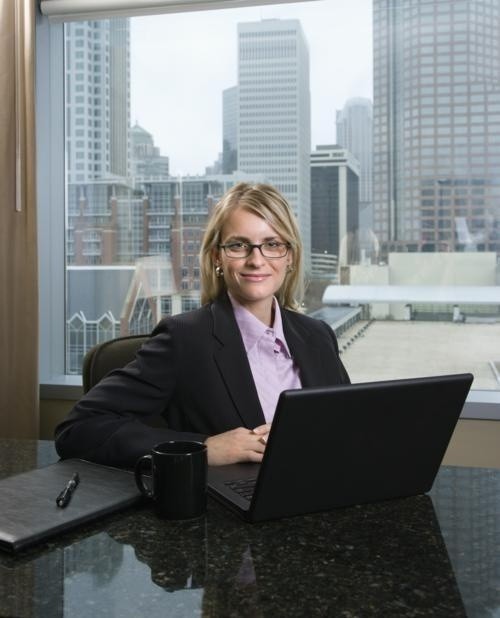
[83,335,171,431]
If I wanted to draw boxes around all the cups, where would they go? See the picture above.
[135,438,207,521]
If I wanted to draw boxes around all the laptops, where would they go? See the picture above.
[201,373,474,517]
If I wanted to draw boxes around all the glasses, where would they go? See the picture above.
[217,239,291,258]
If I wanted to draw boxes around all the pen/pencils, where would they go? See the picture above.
[56,472,79,508]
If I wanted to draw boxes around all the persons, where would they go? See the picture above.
[55,183,352,467]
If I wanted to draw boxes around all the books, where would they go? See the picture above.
[0,458,154,550]
